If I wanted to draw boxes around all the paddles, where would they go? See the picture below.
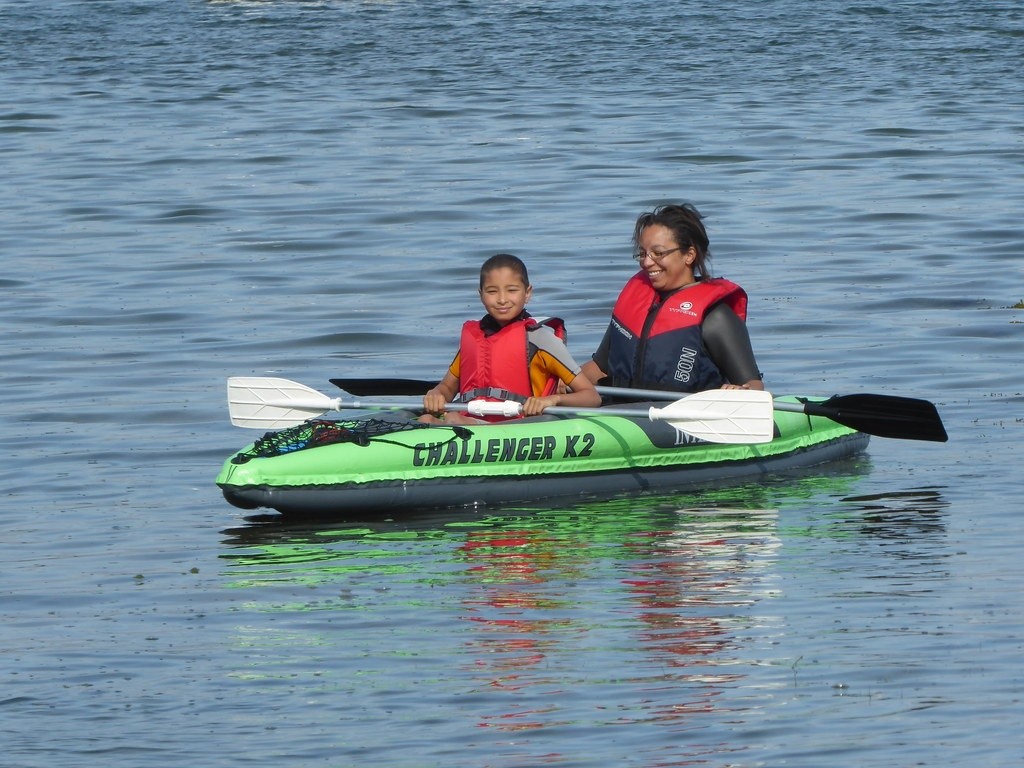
[227,375,948,445]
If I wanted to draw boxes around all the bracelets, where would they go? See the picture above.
[742,383,752,389]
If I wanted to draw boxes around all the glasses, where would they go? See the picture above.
[633,246,690,262]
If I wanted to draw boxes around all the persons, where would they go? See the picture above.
[580,203,764,405]
[418,253,602,425]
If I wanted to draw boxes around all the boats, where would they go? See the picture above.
[210,387,869,523]
